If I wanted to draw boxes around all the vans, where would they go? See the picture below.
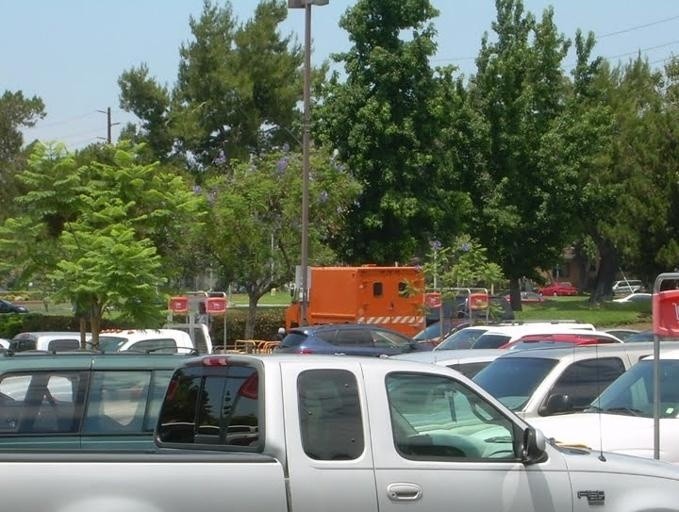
[613,280,644,294]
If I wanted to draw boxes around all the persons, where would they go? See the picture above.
[194,302,213,333]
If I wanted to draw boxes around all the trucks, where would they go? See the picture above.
[282,267,429,337]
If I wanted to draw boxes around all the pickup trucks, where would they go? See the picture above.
[0,351,679,511]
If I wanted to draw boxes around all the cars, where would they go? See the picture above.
[498,290,543,305]
[0,301,29,312]
[273,324,423,359]
[537,282,578,297]
[0,327,197,354]
[386,317,679,423]
[467,350,679,463]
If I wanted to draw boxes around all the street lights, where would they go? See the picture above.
[98,108,120,143]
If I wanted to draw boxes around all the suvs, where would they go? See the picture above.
[0,355,190,447]
